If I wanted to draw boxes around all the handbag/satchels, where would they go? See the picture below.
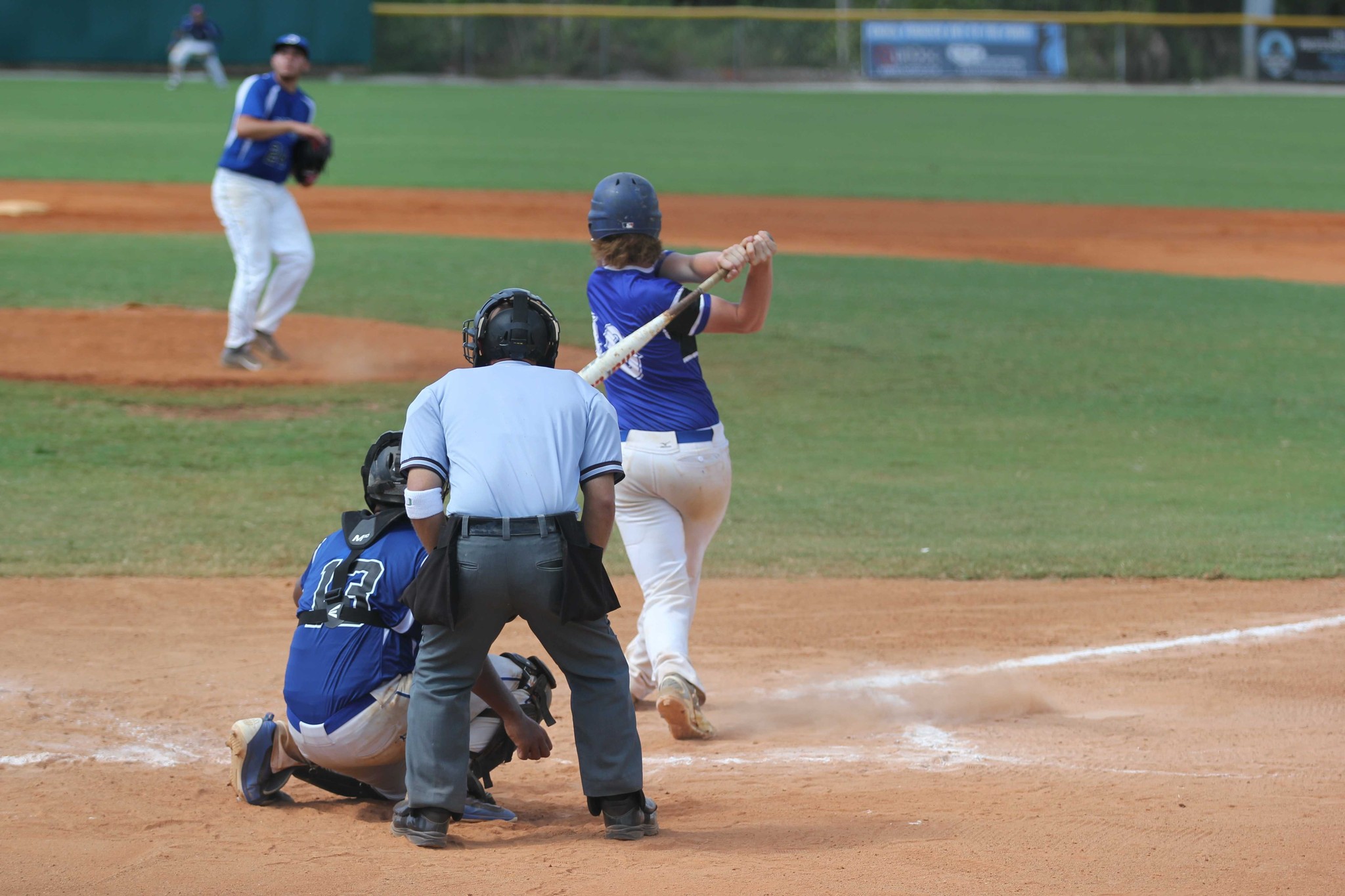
[399,516,458,631]
[557,512,622,625]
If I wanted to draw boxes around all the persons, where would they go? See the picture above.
[211,34,328,371]
[166,4,228,89]
[226,431,556,822]
[391,289,660,849]
[587,171,778,740]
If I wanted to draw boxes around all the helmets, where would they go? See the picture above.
[361,430,405,513]
[473,289,561,369]
[588,171,663,243]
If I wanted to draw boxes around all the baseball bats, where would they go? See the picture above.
[577,244,751,387]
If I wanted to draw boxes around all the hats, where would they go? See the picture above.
[273,34,312,57]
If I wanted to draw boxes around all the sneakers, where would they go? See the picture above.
[463,798,519,825]
[224,713,290,804]
[588,797,660,839]
[655,673,718,741]
[390,798,454,851]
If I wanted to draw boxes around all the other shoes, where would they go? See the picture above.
[255,329,292,362]
[224,349,263,371]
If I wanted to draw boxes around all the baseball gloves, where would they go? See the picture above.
[288,128,333,187]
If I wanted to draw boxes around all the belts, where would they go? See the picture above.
[620,429,714,444]
[456,515,562,536]
